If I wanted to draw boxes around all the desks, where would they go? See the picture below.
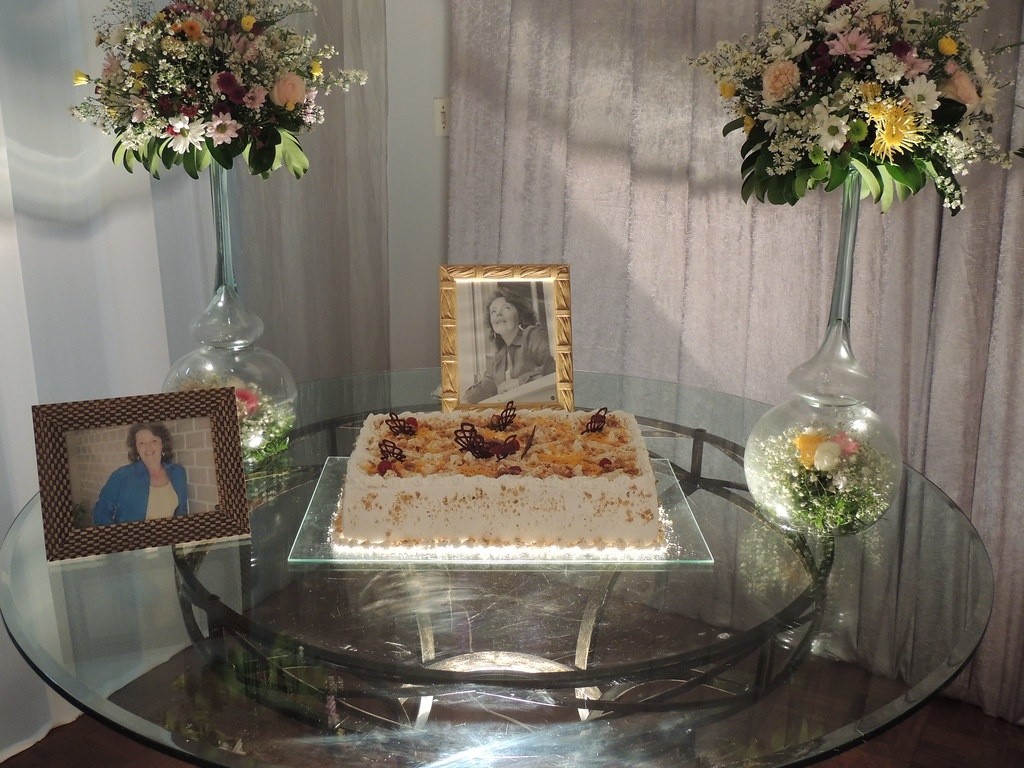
[0,370,994,768]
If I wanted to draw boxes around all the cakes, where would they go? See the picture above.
[329,400,669,556]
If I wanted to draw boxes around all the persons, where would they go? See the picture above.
[458,286,556,405]
[92,422,189,526]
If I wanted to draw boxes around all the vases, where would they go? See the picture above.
[162,154,298,472]
[744,167,902,538]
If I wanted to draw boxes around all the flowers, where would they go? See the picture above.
[69,0,369,180]
[683,0,1024,219]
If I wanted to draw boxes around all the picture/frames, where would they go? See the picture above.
[32,387,250,562]
[440,263,574,413]
